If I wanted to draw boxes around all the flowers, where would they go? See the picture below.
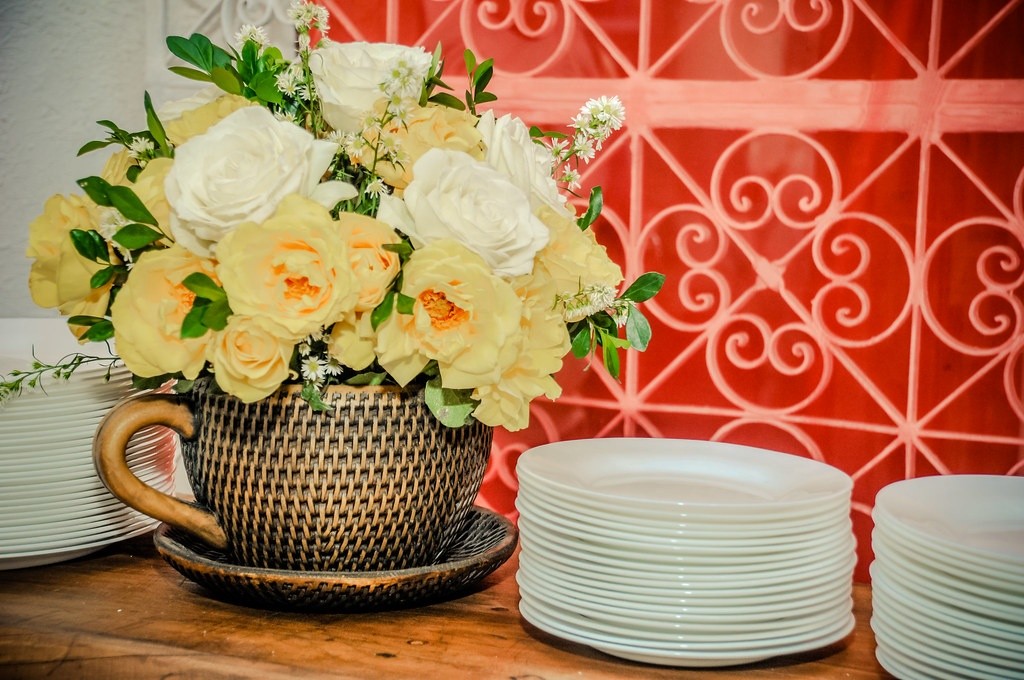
[0,0,667,431]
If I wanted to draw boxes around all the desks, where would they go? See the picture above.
[0,546,887,680]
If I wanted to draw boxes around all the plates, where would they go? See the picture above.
[869,474,1024,680]
[514,437,860,667]
[1,315,177,570]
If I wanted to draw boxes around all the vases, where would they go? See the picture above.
[89,378,494,573]
